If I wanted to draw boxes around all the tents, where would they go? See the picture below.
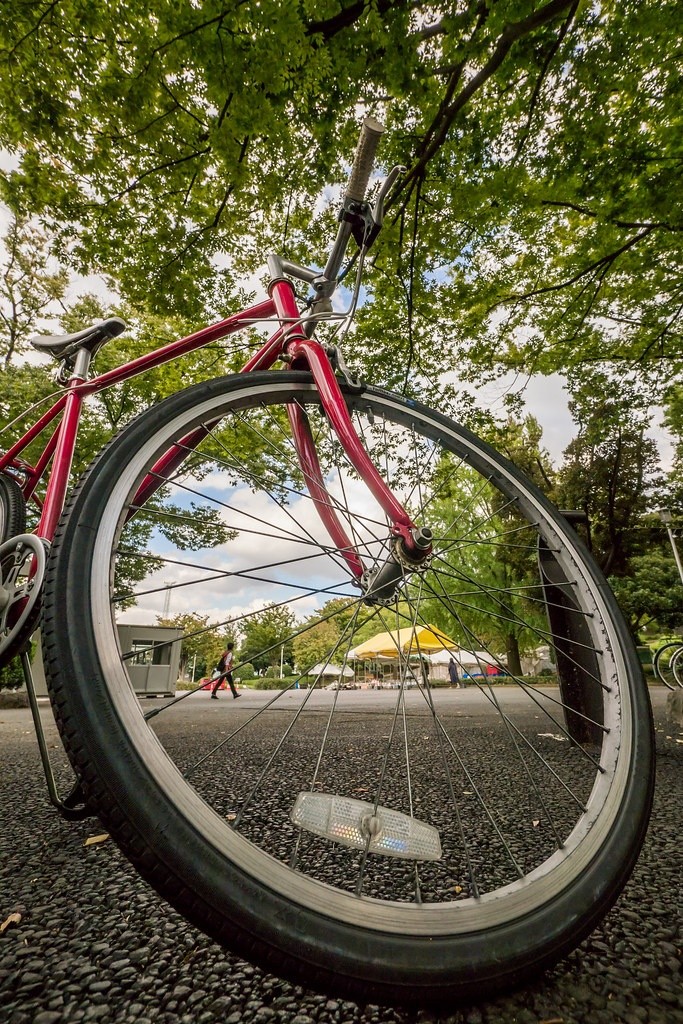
[307,662,356,690]
[519,645,558,679]
[345,624,464,690]
[406,650,510,684]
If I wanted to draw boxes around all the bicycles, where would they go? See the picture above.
[0,119,657,1007]
[652,635,683,691]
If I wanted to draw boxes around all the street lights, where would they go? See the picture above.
[280,644,284,679]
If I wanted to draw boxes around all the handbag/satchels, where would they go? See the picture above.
[217,651,231,671]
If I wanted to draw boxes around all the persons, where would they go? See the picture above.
[211,643,242,699]
[448,658,461,689]
[420,656,430,688]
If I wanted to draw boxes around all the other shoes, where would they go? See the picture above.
[211,695,219,699]
[234,693,241,699]
